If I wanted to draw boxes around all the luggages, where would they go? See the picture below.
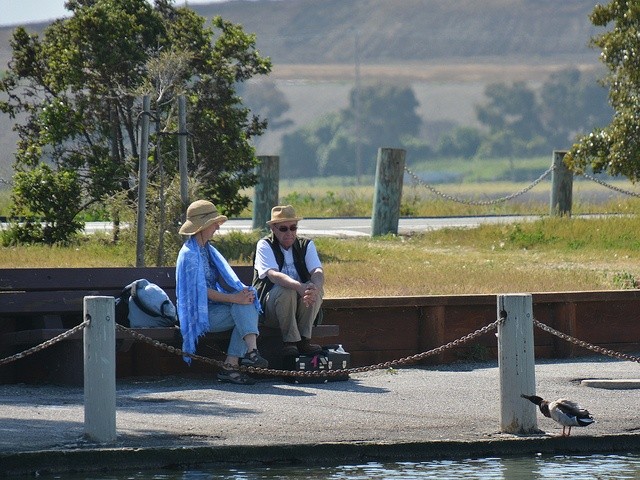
[278,344,351,384]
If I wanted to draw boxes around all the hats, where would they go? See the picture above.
[178,200,228,246]
[266,204,303,226]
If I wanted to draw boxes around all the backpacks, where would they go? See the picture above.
[120,279,176,328]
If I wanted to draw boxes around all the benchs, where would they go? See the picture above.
[0,265,341,345]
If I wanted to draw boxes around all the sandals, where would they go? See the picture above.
[241,349,269,367]
[216,367,256,384]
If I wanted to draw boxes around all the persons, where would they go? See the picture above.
[252,205,325,358]
[176,199,269,385]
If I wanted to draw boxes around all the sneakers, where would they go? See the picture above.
[279,343,297,360]
[299,338,322,353]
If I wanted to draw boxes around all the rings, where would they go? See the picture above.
[250,298,252,302]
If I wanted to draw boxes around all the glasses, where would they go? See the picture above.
[274,225,298,232]
